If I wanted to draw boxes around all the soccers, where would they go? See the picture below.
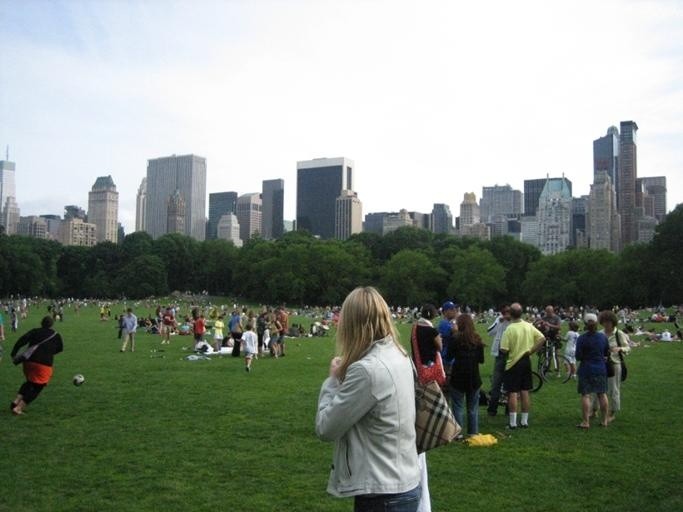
[73,374,85,386]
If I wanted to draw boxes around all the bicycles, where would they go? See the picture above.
[535,333,572,386]
[523,367,542,394]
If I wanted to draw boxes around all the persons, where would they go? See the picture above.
[2,295,342,373]
[11,316,63,415]
[314,286,423,512]
[386,301,682,437]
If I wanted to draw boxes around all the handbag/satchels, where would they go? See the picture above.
[618,355,627,382]
[603,350,616,378]
[407,353,463,456]
[11,330,59,367]
[410,320,447,388]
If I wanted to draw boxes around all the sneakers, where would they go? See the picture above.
[503,422,529,430]
[9,394,28,417]
[244,364,250,372]
[575,421,590,430]
[598,414,617,428]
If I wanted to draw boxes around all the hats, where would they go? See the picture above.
[568,321,579,333]
[441,301,459,311]
[582,312,598,323]
